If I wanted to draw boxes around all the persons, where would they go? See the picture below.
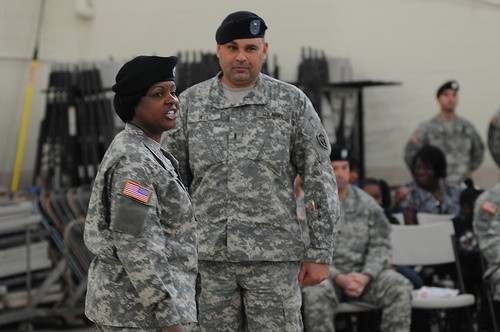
[403,80,485,185]
[293,155,500,332]
[399,144,465,281]
[159,11,340,332]
[83,55,201,332]
[300,143,414,332]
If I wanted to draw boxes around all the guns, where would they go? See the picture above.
[30,46,329,191]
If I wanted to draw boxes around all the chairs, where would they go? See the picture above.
[333,182,498,331]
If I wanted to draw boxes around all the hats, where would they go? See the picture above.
[328,144,351,161]
[112,55,179,95]
[215,11,268,45]
[437,81,459,98]
